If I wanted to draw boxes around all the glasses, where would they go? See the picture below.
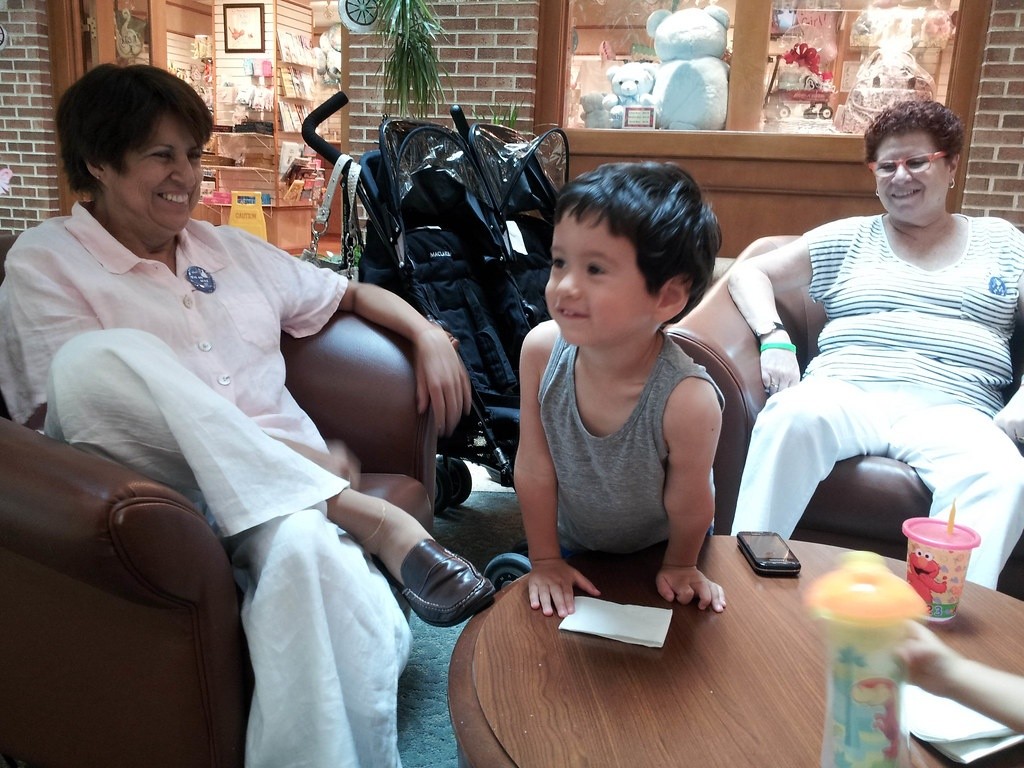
[868,151,949,178]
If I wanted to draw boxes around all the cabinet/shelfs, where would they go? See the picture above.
[82,0,345,252]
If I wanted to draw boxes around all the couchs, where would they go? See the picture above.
[0,231,439,768]
[667,236,1024,601]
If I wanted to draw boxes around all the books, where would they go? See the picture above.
[277,28,326,201]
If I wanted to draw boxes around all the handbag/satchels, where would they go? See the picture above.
[298,154,362,284]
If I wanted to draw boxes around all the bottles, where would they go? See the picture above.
[801,552,929,768]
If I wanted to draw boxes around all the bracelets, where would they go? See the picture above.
[760,342,796,353]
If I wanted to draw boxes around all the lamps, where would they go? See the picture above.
[192,33,212,70]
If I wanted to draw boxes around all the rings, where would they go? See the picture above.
[771,383,778,388]
[1017,437,1024,440]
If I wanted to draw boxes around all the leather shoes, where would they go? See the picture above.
[370,537,496,626]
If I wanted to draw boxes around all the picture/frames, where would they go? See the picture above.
[224,3,265,53]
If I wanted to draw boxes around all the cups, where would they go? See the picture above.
[902,517,981,621]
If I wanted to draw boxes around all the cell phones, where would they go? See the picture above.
[736,531,801,577]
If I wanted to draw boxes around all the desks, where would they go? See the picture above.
[449,536,1024,768]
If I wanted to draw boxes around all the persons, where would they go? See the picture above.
[897,617,1024,768]
[514,159,726,617]
[725,99,1024,589]
[0,64,494,768]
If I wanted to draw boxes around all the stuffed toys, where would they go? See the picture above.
[579,92,609,127]
[646,5,732,130]
[601,61,656,128]
